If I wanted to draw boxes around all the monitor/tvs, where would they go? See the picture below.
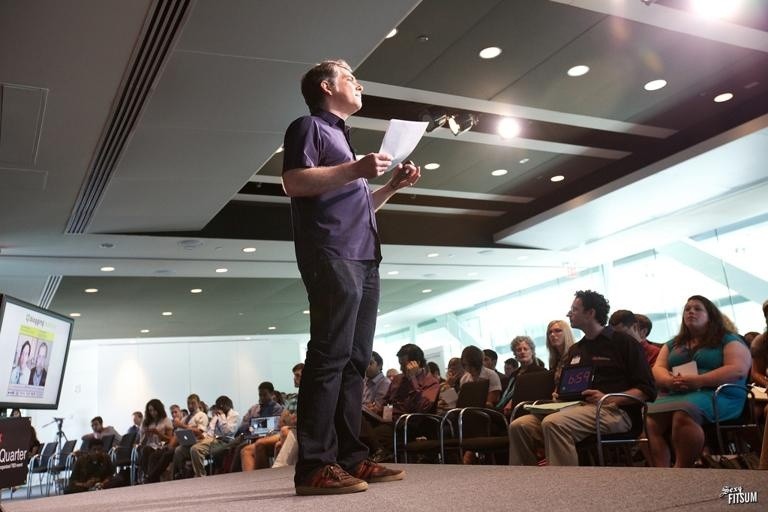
[0,293,74,410]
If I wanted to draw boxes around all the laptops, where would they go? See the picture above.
[175,429,196,446]
[249,416,280,433]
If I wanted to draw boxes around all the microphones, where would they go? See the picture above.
[392,160,414,188]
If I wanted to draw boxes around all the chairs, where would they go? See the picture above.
[25,352,767,498]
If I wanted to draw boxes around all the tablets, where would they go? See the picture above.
[559,364,596,393]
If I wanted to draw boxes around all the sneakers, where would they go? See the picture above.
[353,457,406,484]
[295,463,368,495]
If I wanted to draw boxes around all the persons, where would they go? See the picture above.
[29,342,48,386]
[9,409,21,418]
[65,287,767,494]
[9,340,30,385]
[277,54,424,496]
[27,424,40,465]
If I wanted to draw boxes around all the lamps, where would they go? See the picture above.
[447,111,482,136]
[421,111,449,133]
